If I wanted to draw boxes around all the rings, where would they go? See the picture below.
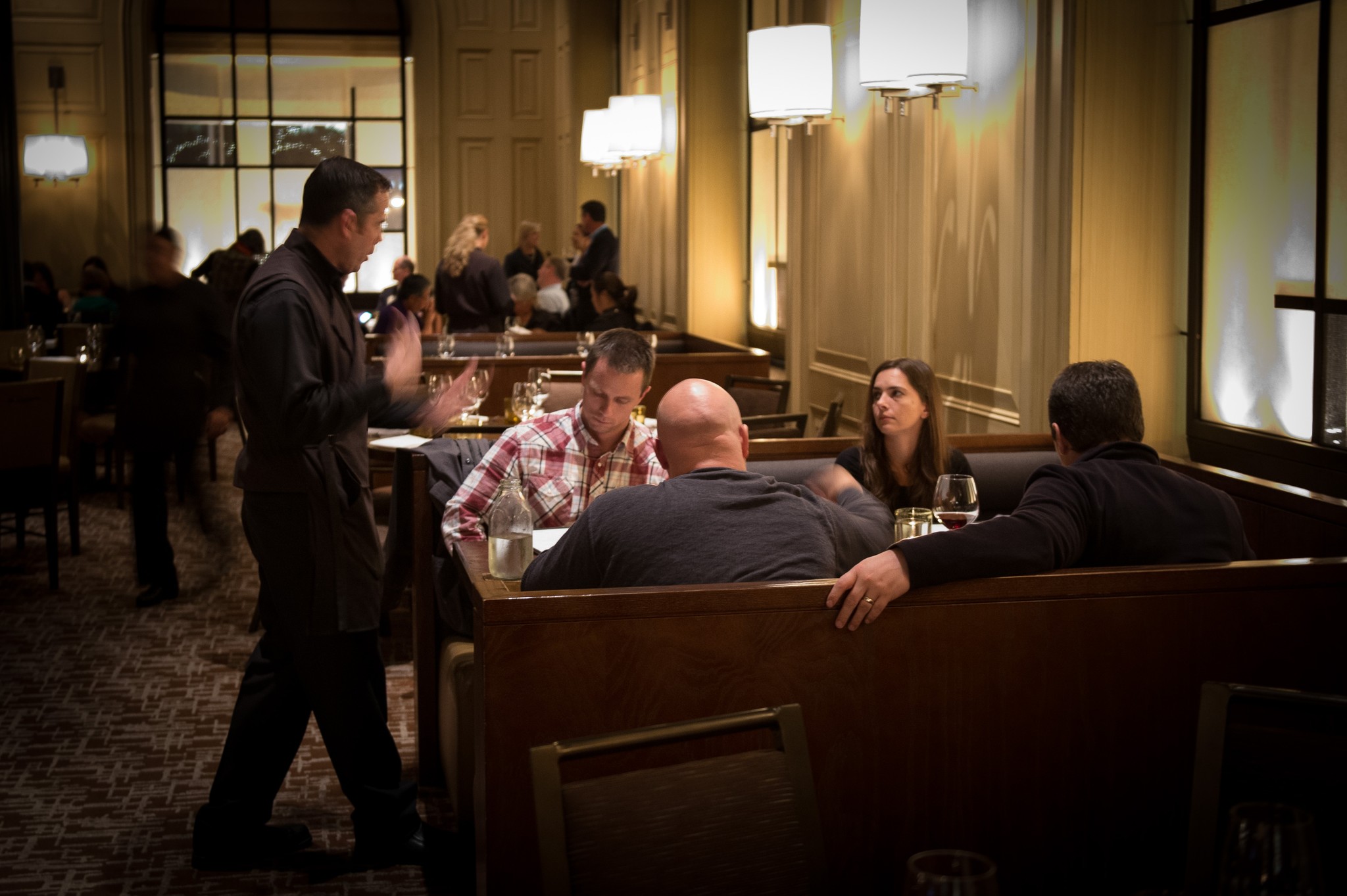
[863,596,874,606]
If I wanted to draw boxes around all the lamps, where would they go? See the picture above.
[580,109,623,177]
[747,24,846,140]
[607,94,663,168]
[859,0,979,117]
[23,66,90,189]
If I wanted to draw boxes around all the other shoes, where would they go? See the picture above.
[353,819,427,864]
[135,580,179,608]
[191,822,312,873]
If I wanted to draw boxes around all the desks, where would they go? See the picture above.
[456,531,530,601]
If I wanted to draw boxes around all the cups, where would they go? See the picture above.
[575,330,595,355]
[894,506,932,539]
[428,366,491,427]
[934,474,980,529]
[438,336,455,358]
[495,335,514,356]
[511,366,554,423]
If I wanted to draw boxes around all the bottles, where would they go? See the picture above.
[487,479,533,580]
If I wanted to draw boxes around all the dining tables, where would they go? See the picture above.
[473,554,1347,895]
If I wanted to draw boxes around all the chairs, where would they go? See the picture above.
[525,361,847,438]
[0,321,235,594]
[531,702,838,896]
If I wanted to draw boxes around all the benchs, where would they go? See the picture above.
[359,327,772,413]
[406,428,1063,896]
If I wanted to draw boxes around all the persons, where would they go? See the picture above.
[373,197,639,371]
[23,253,116,327]
[825,359,1248,631]
[188,229,267,421]
[443,327,670,560]
[193,155,430,885]
[97,223,243,613]
[836,356,976,513]
[518,377,896,592]
